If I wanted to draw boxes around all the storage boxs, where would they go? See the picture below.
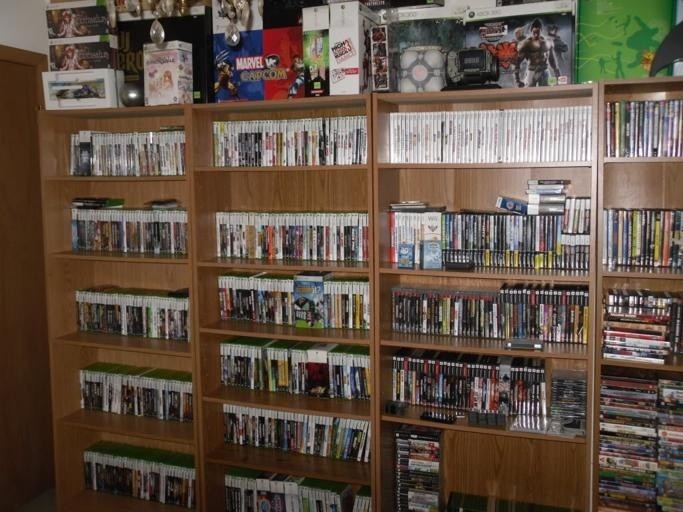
[39,0,195,112]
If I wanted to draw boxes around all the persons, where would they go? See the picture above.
[513,17,569,88]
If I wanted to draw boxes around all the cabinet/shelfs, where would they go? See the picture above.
[37,92,194,509]
[197,92,375,512]
[371,78,596,512]
[597,75,682,512]
